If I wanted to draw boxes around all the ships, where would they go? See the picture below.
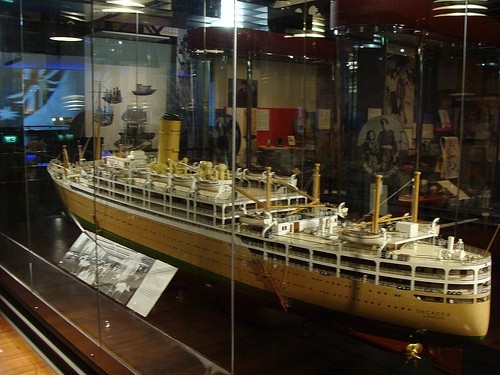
[45,112,491,338]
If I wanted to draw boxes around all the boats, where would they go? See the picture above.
[94,83,157,152]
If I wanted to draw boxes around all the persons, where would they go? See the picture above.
[377,118,398,171]
[362,129,378,169]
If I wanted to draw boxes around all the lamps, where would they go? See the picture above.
[284,6,326,38]
[431,1,488,19]
[45,2,85,45]
[353,26,386,49]
